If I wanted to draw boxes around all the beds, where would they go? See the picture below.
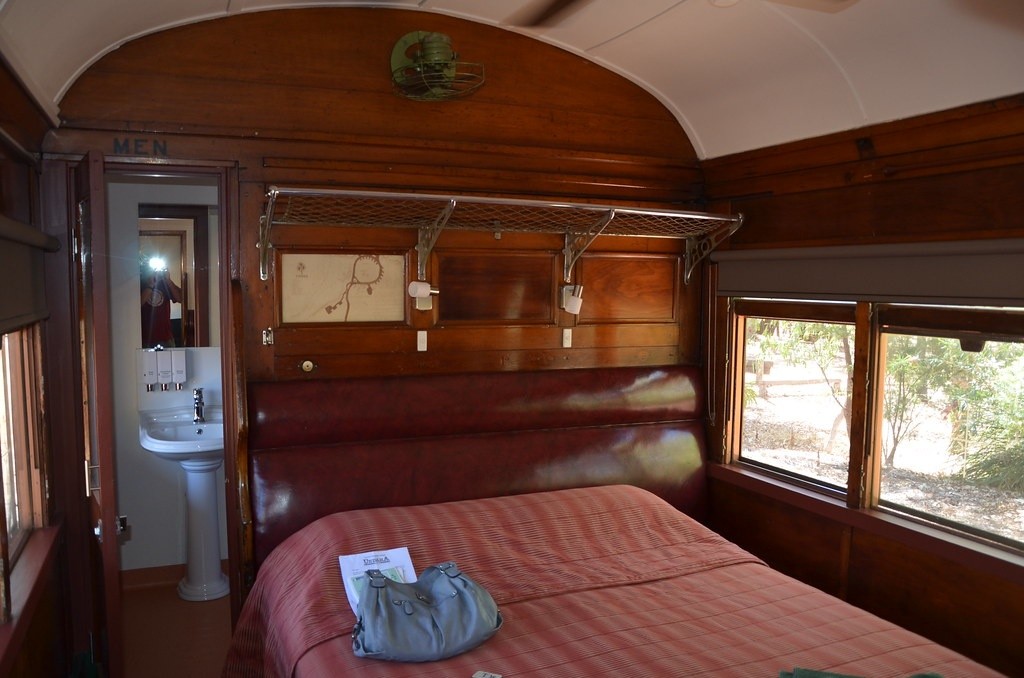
[230,485,1009,677]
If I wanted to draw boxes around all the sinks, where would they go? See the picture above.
[138,418,224,461]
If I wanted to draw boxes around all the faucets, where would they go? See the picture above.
[192,387,207,424]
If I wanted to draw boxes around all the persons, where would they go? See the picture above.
[141,259,183,349]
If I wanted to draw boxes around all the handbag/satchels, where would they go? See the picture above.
[352,561,503,662]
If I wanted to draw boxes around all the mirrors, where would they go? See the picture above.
[137,202,222,347]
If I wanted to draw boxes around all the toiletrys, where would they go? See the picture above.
[156,350,172,392]
[171,350,187,391]
[142,351,157,393]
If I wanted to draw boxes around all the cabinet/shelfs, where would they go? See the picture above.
[256,183,747,290]
